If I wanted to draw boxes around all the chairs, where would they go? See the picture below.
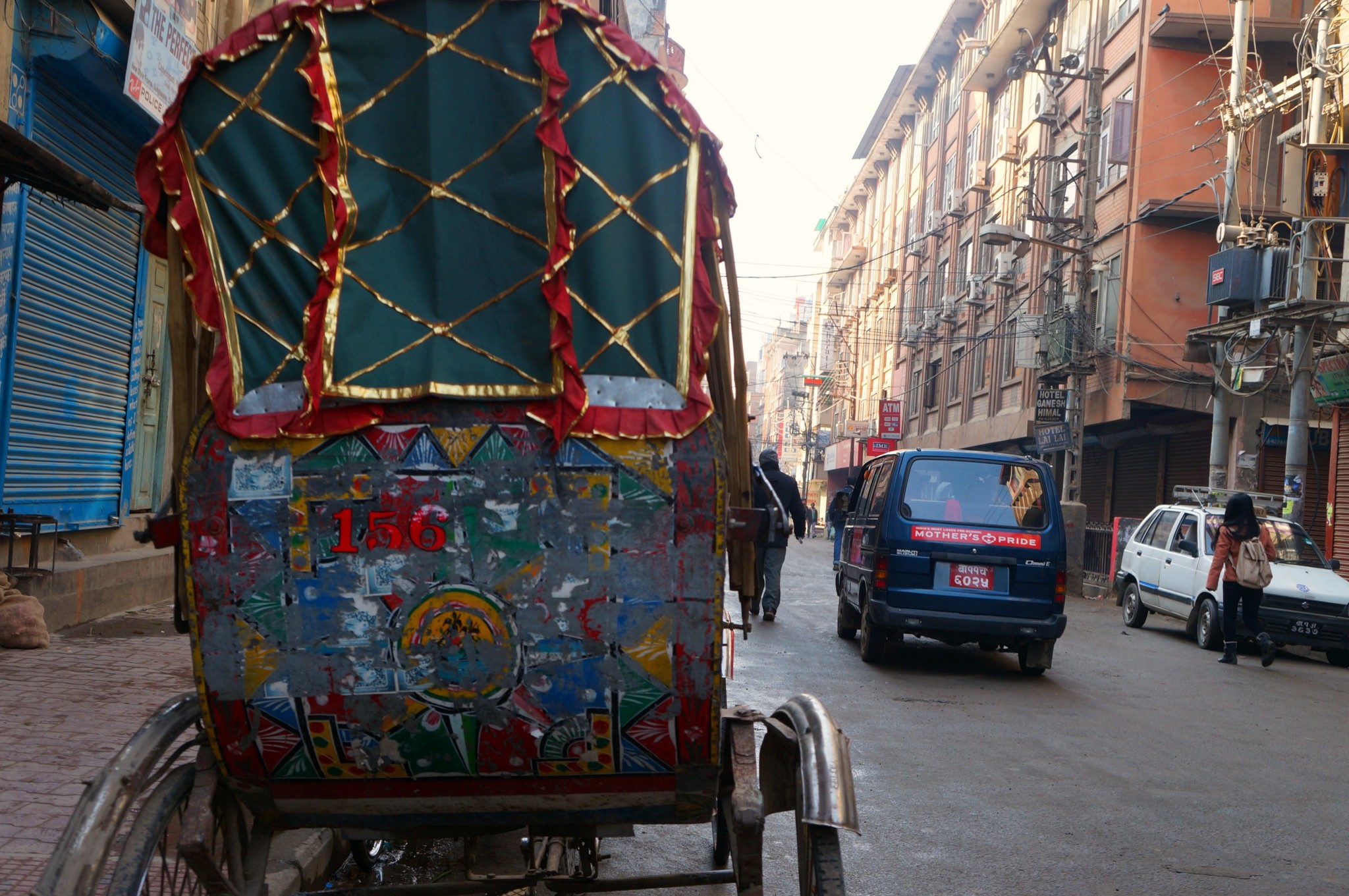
[1181,522,1197,555]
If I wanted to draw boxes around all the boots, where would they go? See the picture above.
[1218,639,1238,664]
[1256,632,1274,667]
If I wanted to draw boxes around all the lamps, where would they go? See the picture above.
[960,37,988,51]
[830,256,843,262]
[853,428,878,435]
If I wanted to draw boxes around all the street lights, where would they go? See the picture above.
[979,226,1091,501]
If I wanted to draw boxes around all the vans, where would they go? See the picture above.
[835,447,1068,674]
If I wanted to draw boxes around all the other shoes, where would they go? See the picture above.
[812,535,817,539]
[748,602,761,615]
[762,607,775,620]
[833,564,839,570]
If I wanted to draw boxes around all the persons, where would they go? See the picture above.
[807,503,818,538]
[825,506,833,540]
[749,449,806,621]
[801,499,808,539]
[829,476,857,571]
[1205,493,1276,668]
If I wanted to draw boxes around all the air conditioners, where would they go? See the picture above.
[1035,89,1057,114]
[909,233,923,252]
[942,294,956,317]
[927,209,942,233]
[969,160,987,189]
[995,126,1018,158]
[993,251,1013,282]
[833,267,896,337]
[922,308,937,328]
[904,323,918,341]
[948,188,963,213]
[835,423,844,437]
[966,274,982,302]
[867,420,878,436]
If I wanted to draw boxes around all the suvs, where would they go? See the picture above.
[1115,484,1349,669]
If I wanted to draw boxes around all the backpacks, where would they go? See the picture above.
[1226,524,1273,589]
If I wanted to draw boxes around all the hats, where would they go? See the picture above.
[758,450,779,463]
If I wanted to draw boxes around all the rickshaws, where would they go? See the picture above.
[27,0,861,896]
